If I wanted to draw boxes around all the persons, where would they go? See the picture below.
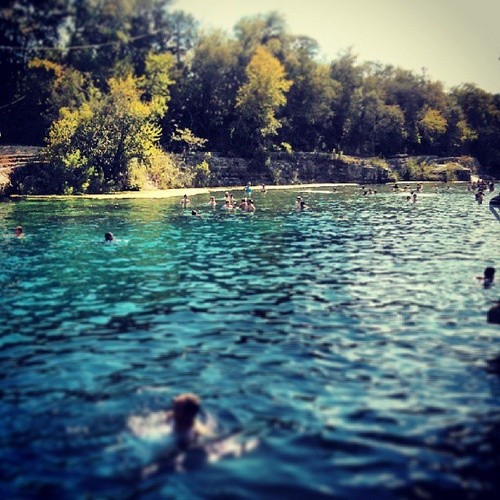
[180,195,191,205]
[165,392,214,439]
[208,182,257,213]
[361,185,377,197]
[295,197,308,212]
[104,232,114,241]
[15,227,23,238]
[191,210,203,220]
[467,178,494,204]
[392,182,423,202]
[261,184,267,192]
[433,184,459,191]
[332,187,338,193]
[484,267,496,282]
